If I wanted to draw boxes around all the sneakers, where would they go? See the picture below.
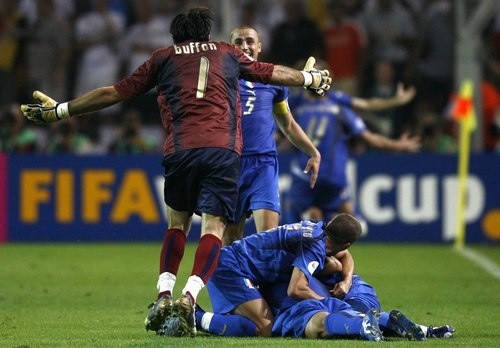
[173,295,197,337]
[386,309,425,340]
[428,324,455,340]
[360,308,385,342]
[143,295,173,334]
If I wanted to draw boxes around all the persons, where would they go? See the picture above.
[274,61,416,215]
[276,86,422,223]
[19,6,332,342]
[194,213,454,341]
[221,25,321,247]
[0,0,500,157]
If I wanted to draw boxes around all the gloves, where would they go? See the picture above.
[20,91,71,126]
[299,57,332,97]
